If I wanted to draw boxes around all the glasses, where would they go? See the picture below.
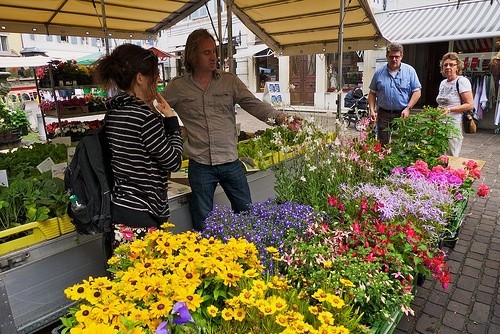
[443,62,458,67]
[140,48,157,62]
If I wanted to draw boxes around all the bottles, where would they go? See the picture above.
[69,194,86,213]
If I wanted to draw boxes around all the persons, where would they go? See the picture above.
[367,42,422,146]
[159,29,302,232]
[91,42,183,282]
[435,51,475,157]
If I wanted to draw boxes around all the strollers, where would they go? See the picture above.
[340,86,369,131]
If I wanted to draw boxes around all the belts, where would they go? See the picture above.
[379,106,401,113]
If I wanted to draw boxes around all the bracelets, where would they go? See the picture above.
[446,106,451,114]
[406,105,413,111]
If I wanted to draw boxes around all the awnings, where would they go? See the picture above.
[168,39,234,53]
[231,45,277,58]
[365,0,500,45]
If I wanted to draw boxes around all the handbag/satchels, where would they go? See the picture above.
[455,77,477,134]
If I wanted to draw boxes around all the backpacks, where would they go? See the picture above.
[64,128,112,236]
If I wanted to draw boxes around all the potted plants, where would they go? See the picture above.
[0,60,488,334]
